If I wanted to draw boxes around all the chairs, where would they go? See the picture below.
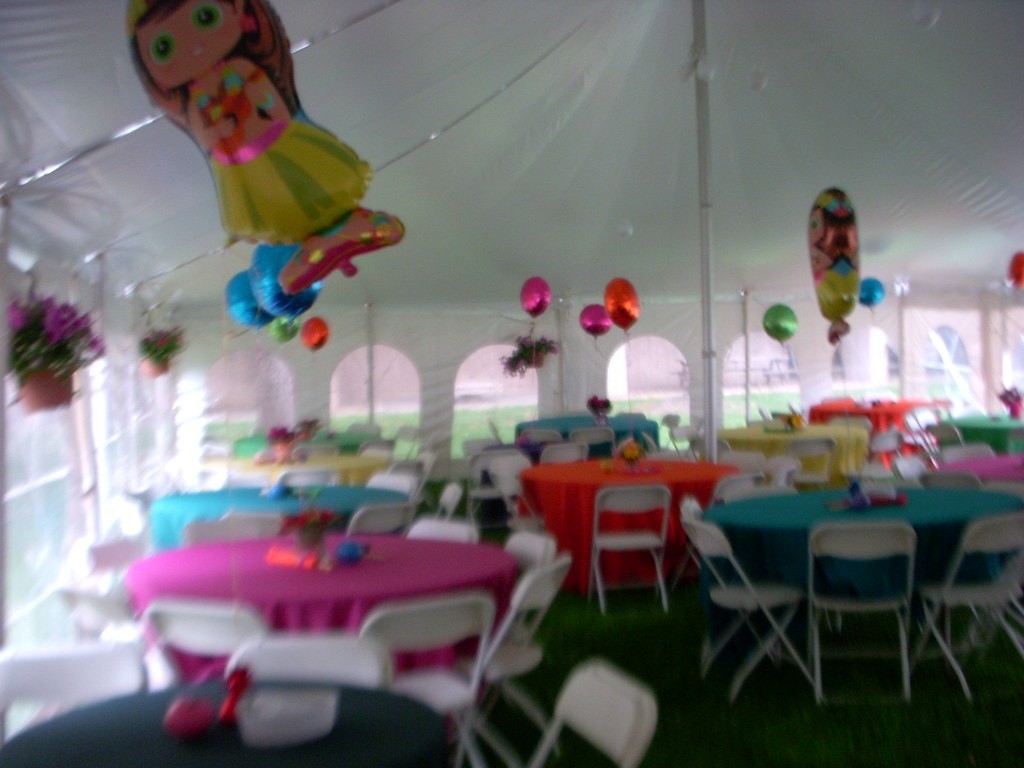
[0,422,1024,768]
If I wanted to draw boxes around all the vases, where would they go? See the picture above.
[302,427,311,438]
[526,350,548,367]
[274,441,296,464]
[594,408,607,427]
[1008,400,1021,417]
[22,368,76,409]
[142,353,169,378]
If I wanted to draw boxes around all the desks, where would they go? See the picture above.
[0,686,456,768]
[0,394,1023,675]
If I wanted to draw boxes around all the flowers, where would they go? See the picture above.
[503,333,558,374]
[4,295,107,373]
[297,420,316,429]
[1000,386,1020,406]
[588,395,612,413]
[136,323,189,358]
[266,424,296,445]
